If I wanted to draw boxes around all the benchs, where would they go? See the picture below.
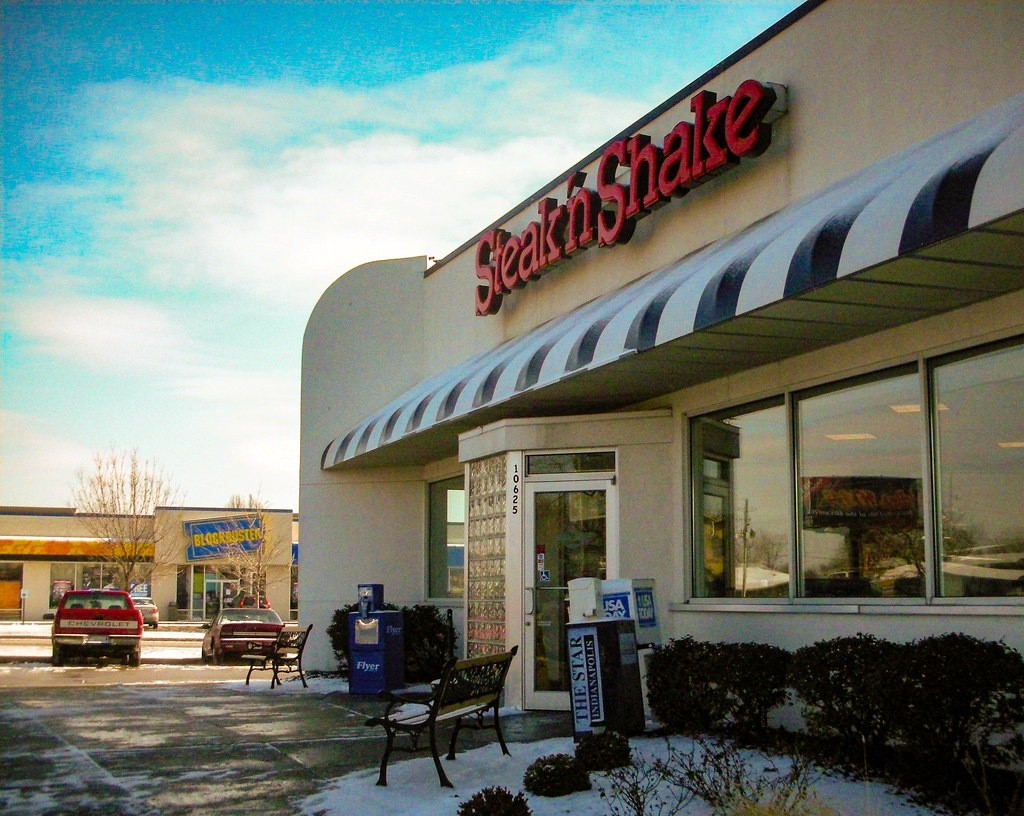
[365,644,518,790]
[243,623,315,688]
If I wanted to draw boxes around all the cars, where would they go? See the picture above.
[131,597,159,628]
[227,594,271,609]
[201,607,288,665]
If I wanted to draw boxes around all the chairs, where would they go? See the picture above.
[108,605,121,609]
[70,604,84,608]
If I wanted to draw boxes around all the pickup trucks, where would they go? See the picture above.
[43,589,143,667]
